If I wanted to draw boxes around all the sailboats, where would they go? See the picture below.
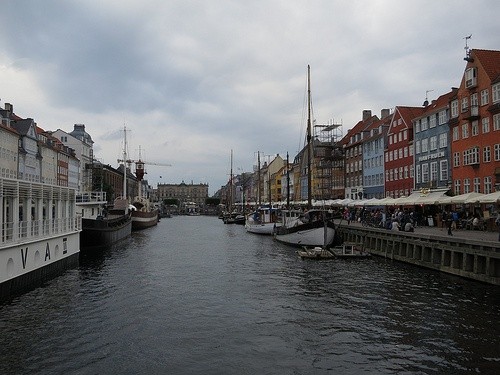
[126,160,159,228]
[222,149,301,233]
[273,65,336,248]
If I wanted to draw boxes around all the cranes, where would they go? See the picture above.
[117,145,172,166]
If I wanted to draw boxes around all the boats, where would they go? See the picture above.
[297,245,336,260]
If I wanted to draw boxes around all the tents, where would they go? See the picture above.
[232,190,500,205]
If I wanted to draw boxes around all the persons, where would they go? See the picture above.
[298,206,500,232]
